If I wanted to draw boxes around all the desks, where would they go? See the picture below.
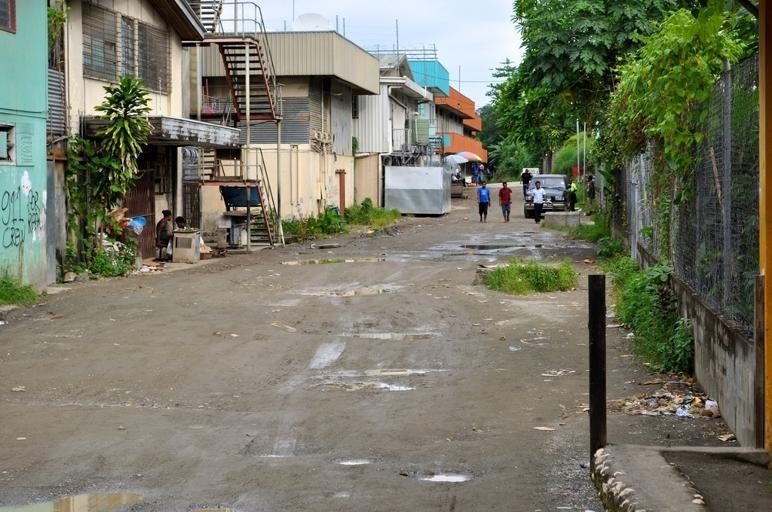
[172,227,202,264]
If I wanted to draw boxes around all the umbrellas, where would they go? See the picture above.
[444,155,469,164]
[456,151,482,161]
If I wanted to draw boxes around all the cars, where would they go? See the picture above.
[523,174,571,219]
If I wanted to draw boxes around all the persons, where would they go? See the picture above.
[175,216,204,247]
[521,169,532,197]
[452,173,466,185]
[564,178,578,211]
[498,182,512,223]
[156,210,173,259]
[570,175,573,183]
[527,181,547,224]
[586,175,595,208]
[477,186,490,223]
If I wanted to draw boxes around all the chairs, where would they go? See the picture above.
[152,229,173,263]
